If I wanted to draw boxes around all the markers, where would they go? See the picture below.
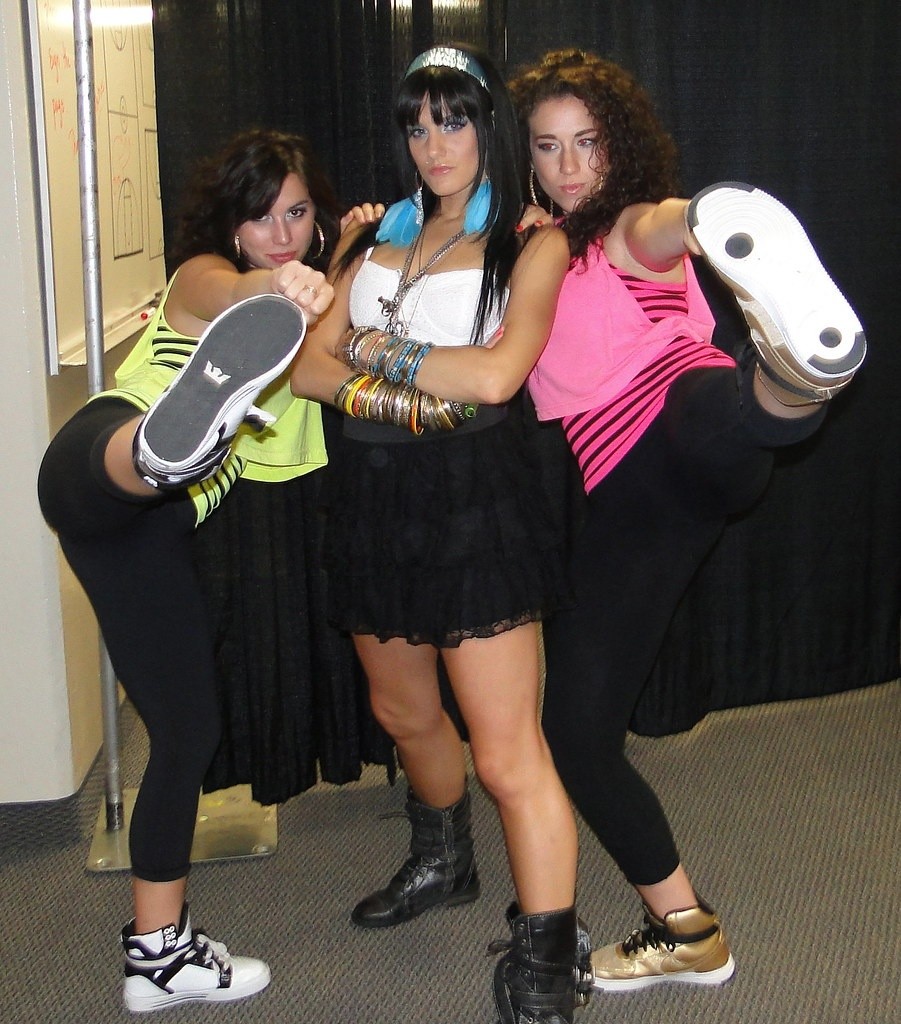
[141,306,156,320]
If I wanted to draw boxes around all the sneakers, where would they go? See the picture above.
[123,901,273,1013]
[687,178,867,411]
[132,295,307,486]
[587,892,737,992]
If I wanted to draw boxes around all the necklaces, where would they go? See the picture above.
[378,206,465,336]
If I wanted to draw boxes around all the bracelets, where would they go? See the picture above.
[332,372,482,437]
[341,324,436,388]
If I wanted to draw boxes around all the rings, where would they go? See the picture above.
[303,284,318,297]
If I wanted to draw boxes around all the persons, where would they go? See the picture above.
[35,130,388,1015]
[288,43,589,1024]
[503,48,867,996]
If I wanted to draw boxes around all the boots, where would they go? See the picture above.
[350,782,480,926]
[491,900,580,1023]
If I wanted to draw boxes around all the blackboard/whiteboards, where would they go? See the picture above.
[26,0,167,375]
